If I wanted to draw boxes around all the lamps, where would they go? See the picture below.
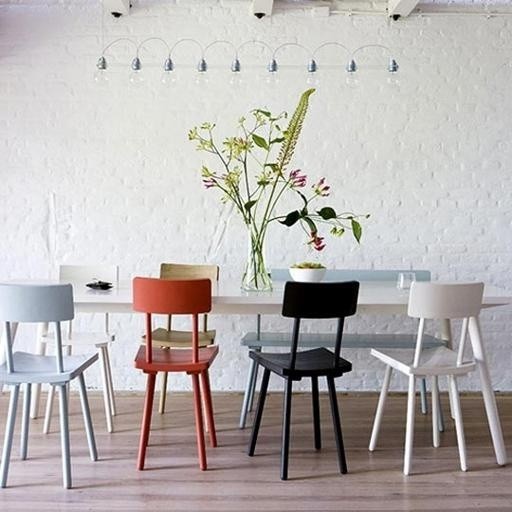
[91,34,404,73]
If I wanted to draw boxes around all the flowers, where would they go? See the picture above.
[184,87,373,288]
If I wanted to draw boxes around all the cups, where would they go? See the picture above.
[396,272,416,289]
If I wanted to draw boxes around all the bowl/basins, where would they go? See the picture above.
[289,263,326,283]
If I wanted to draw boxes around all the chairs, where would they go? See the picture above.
[239,266,447,437]
[47,262,118,434]
[241,280,361,483]
[130,276,219,473]
[1,282,102,490]
[144,261,219,417]
[368,279,486,477]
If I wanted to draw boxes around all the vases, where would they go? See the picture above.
[240,220,274,295]
